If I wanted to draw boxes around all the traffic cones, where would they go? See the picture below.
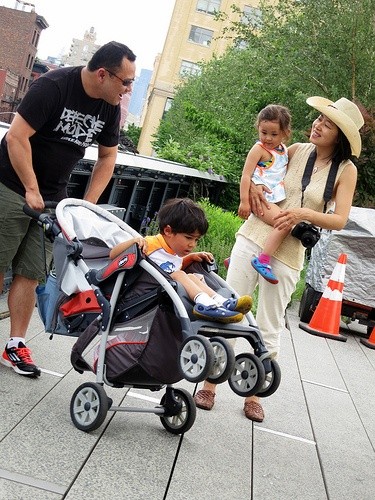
[360,325,375,349]
[298,254,348,343]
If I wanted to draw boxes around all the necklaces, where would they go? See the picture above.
[312,154,334,174]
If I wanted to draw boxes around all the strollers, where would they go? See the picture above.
[23,198,281,434]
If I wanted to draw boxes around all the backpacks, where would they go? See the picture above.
[35,222,96,337]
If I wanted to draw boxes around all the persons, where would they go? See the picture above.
[224,104,292,287]
[110,198,253,323]
[194,96,365,422]
[1,41,136,377]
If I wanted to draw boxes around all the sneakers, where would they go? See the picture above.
[0,341,42,377]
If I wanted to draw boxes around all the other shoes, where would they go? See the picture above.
[192,303,244,324]
[223,256,231,270]
[194,389,216,410]
[243,399,265,423]
[251,257,279,284]
[222,294,254,316]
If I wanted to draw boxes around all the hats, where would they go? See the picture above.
[306,95,365,158]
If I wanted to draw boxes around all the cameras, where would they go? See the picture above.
[290,222,320,249]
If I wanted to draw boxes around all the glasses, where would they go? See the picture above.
[103,66,135,87]
